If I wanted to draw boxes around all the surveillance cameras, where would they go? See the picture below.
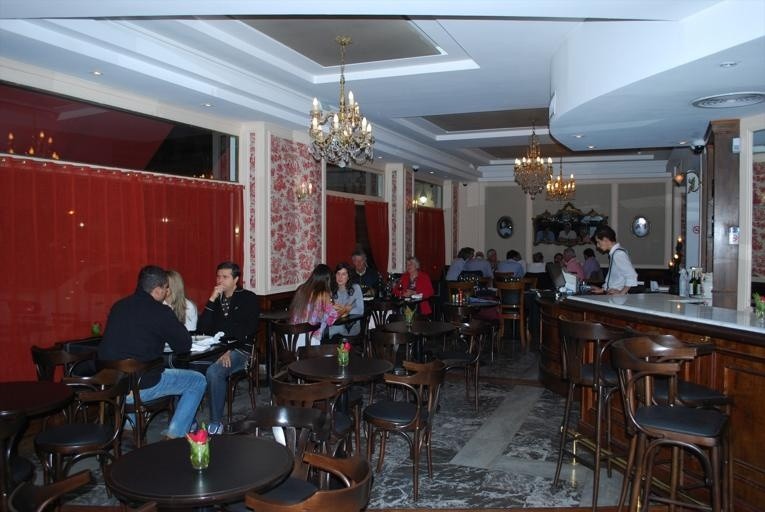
[692,139,704,152]
[412,165,420,172]
[462,182,467,186]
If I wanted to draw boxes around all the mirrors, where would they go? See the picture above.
[532,203,609,248]
[632,213,651,239]
[496,215,514,239]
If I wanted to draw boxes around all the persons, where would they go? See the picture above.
[668,241,682,295]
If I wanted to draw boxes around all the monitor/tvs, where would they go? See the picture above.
[545,261,566,292]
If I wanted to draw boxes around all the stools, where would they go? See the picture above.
[553,315,640,512]
[623,328,730,512]
[611,335,734,512]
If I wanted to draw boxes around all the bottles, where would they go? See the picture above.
[376,272,404,302]
[688,270,702,299]
[451,288,463,303]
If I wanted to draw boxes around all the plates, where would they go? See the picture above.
[192,335,213,342]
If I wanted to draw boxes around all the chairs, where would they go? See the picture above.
[440,269,554,413]
[1,263,443,512]
[586,267,680,296]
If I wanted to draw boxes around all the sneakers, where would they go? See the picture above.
[207,421,224,435]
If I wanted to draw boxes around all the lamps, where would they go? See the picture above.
[305,32,376,170]
[513,118,553,199]
[420,181,427,203]
[545,151,579,203]
[673,169,702,195]
